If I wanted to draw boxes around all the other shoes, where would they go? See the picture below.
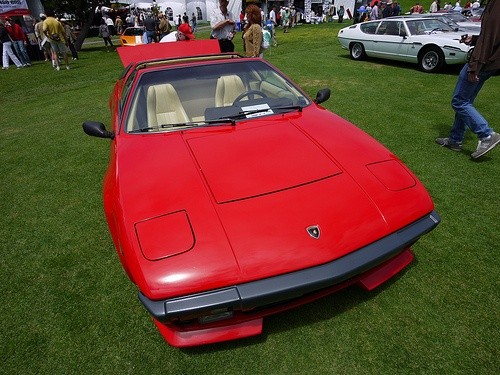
[66,66,71,69]
[56,67,61,71]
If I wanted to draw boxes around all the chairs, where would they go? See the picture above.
[386,23,398,34]
[214,75,246,107]
[146,83,191,130]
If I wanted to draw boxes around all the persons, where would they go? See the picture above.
[209,0,481,59]
[435,0,500,160]
[98,7,198,53]
[0,13,80,70]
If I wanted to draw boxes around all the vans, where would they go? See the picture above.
[0,9,41,61]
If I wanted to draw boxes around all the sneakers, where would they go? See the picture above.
[435,138,462,151]
[471,132,500,159]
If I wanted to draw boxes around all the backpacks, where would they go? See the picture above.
[262,30,271,49]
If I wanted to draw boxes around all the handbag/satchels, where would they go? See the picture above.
[52,33,61,42]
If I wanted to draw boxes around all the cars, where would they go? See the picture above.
[119,26,150,47]
[82,38,441,349]
[336,7,487,73]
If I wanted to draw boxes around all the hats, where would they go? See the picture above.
[40,13,45,17]
[178,23,195,39]
[60,18,66,22]
